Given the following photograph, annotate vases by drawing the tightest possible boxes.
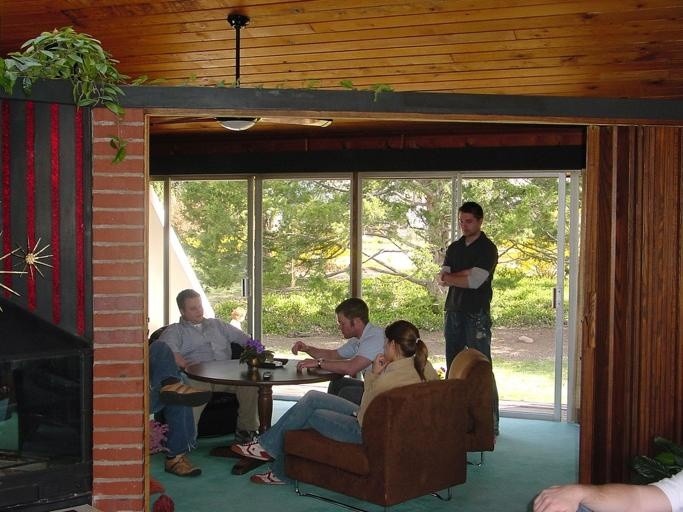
[249,358,259,366]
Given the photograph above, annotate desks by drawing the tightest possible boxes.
[184,358,344,475]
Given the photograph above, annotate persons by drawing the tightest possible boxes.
[157,289,261,444]
[149,340,212,477]
[531,469,682,512]
[229,320,441,485]
[291,298,385,405]
[438,202,499,436]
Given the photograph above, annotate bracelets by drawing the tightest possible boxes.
[442,272,449,281]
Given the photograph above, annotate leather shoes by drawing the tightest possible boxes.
[235,431,259,444]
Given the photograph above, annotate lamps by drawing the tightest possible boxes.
[214,117,261,131]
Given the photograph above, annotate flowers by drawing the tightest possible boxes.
[239,339,275,364]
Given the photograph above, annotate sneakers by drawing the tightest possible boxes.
[160,382,212,407]
[250,470,287,484]
[165,453,201,476]
[231,437,270,461]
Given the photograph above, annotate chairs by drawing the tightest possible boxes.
[283,379,468,511]
[149,326,239,438]
[447,349,496,466]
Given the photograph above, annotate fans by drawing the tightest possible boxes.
[150,116,334,129]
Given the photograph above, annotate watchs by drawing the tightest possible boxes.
[317,358,324,368]
